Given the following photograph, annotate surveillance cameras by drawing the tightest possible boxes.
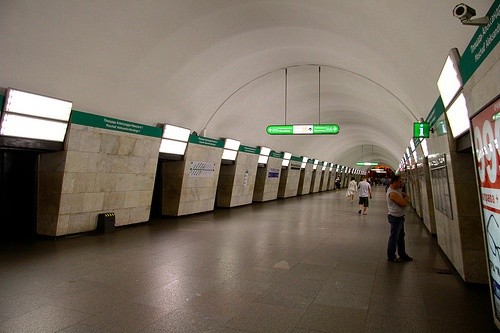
[452,3,477,20]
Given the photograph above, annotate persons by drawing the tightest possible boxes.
[367,177,385,186]
[347,178,358,203]
[386,175,413,262]
[335,176,341,194]
[357,176,372,216]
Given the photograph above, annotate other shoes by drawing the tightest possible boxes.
[358,210,361,214]
[363,213,367,215]
[399,255,413,261]
[387,258,402,264]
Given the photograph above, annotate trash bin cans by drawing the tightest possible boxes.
[97,213,115,235]
